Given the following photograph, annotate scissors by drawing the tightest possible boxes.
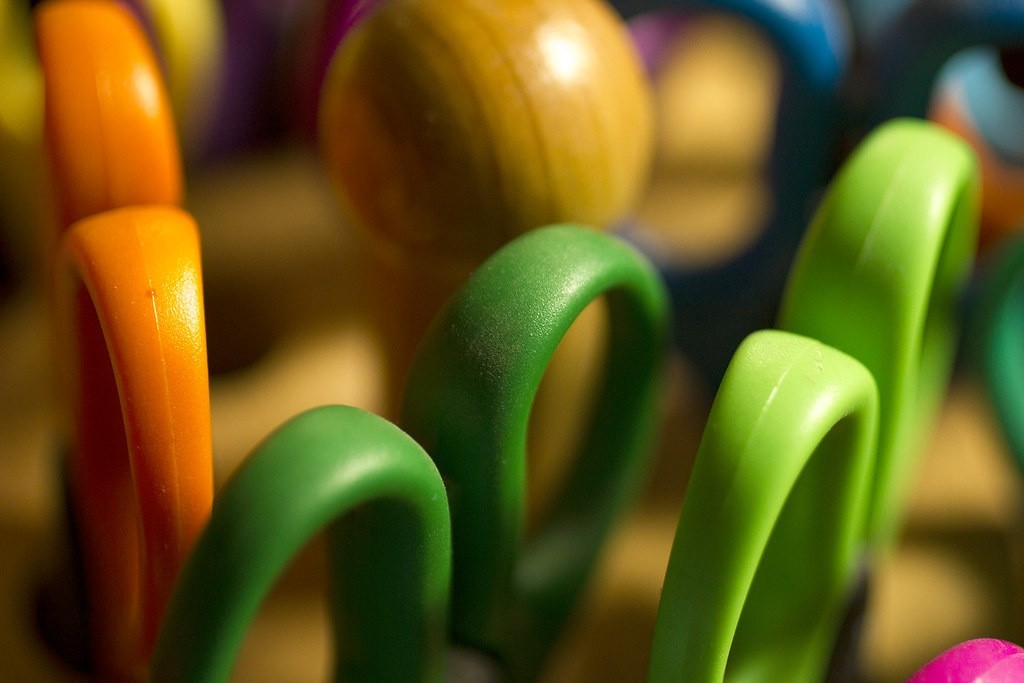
[30,0,218,683]
[149,223,675,683]
[647,122,985,683]
